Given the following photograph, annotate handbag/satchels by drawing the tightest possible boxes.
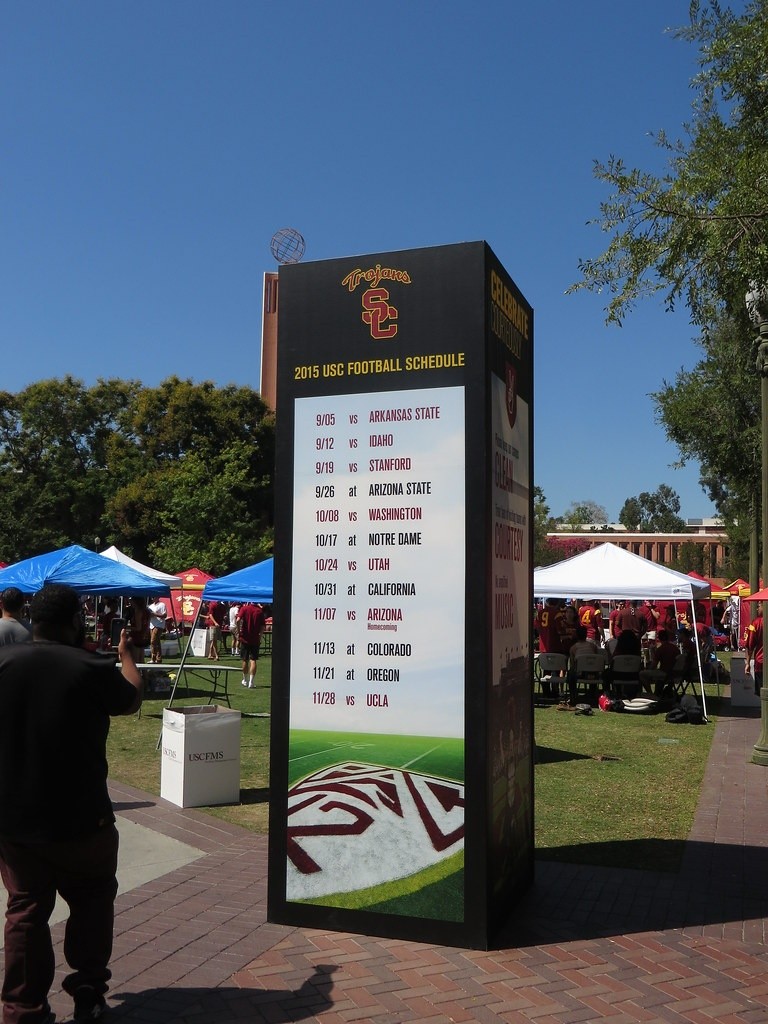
[665,704,708,724]
[711,663,731,685]
[132,632,151,648]
[598,691,627,713]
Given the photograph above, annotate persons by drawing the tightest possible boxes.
[197,600,271,656]
[77,596,135,648]
[0,584,145,1024]
[125,596,149,674]
[236,602,265,689]
[208,601,227,661]
[535,597,739,699]
[0,587,32,647]
[146,595,167,663]
[745,601,764,697]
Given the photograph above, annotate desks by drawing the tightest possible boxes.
[115,663,245,719]
[220,630,272,653]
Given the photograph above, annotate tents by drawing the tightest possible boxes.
[98,545,184,655]
[0,545,190,693]
[155,557,273,749]
[158,568,242,623]
[654,571,768,653]
[534,541,720,723]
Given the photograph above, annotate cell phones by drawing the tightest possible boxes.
[111,618,126,648]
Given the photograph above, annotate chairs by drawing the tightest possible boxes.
[659,655,686,705]
[609,654,641,699]
[676,655,702,704]
[574,654,605,699]
[538,653,568,702]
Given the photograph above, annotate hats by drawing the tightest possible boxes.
[681,693,701,709]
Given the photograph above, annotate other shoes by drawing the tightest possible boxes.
[208,656,213,658]
[231,654,235,656]
[248,684,256,688]
[147,659,155,663]
[216,656,220,661]
[73,996,106,1021]
[234,653,240,656]
[155,660,162,664]
[241,680,248,686]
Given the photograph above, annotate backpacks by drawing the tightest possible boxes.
[575,703,593,715]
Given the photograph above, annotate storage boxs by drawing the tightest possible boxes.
[149,676,171,692]
[144,642,178,657]
[160,704,242,809]
[190,629,211,657]
